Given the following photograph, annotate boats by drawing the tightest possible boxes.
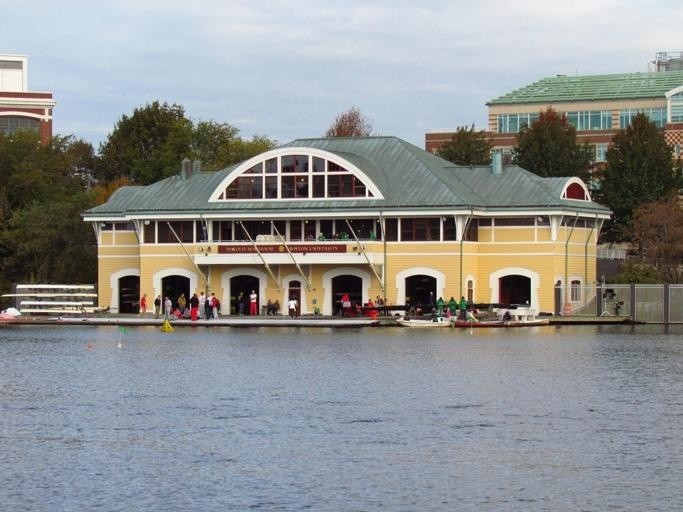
[393,315,452,327]
[495,307,551,327]
[453,318,505,328]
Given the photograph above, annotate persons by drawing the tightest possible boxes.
[449,297,457,316]
[316,232,325,241]
[341,293,351,307]
[428,291,436,312]
[238,291,245,316]
[250,290,258,315]
[334,231,349,241]
[367,229,375,241]
[304,230,314,241]
[138,294,148,319]
[459,296,468,321]
[266,300,280,316]
[501,310,513,327]
[288,296,298,318]
[368,295,384,307]
[155,288,220,320]
[438,297,445,313]
